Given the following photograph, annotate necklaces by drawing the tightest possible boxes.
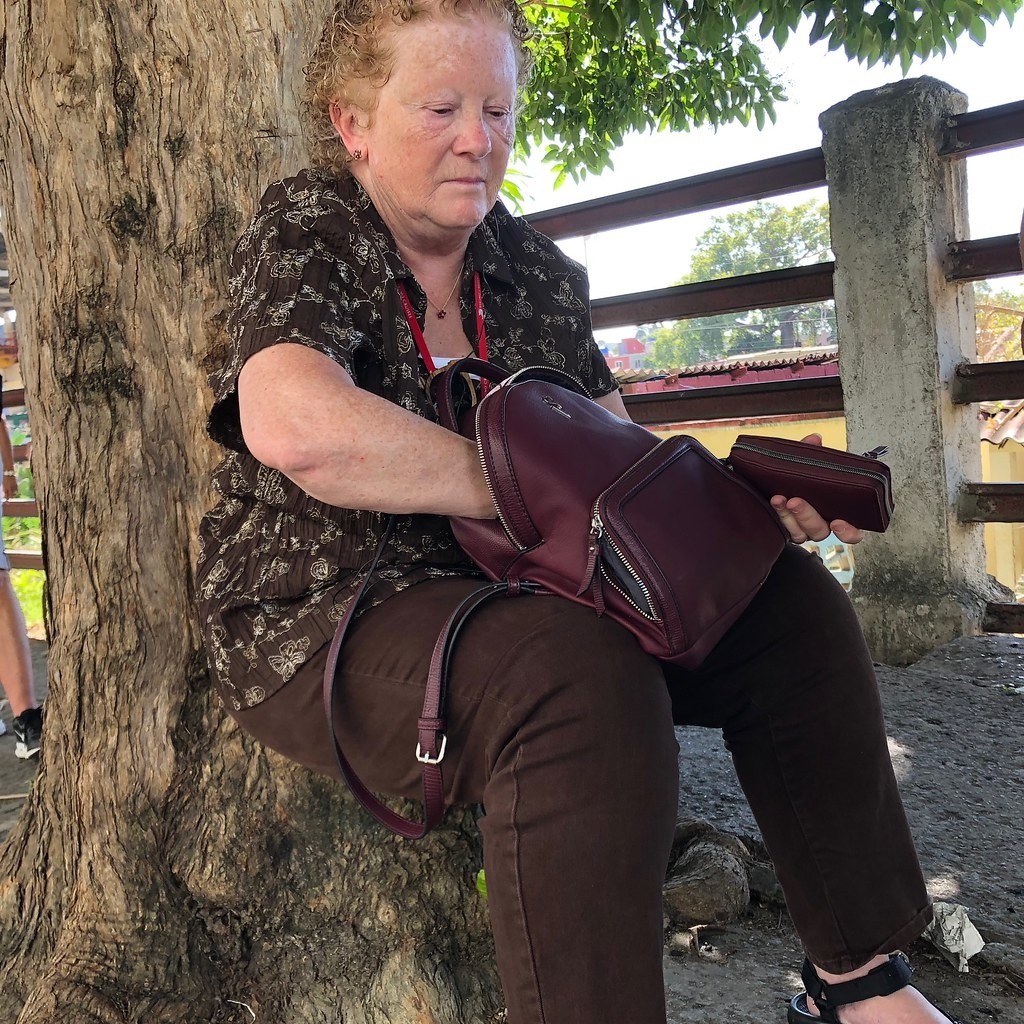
[426,258,466,318]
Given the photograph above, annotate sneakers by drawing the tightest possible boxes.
[0,719,6,736]
[12,704,44,759]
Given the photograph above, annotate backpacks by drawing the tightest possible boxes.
[330,361,789,836]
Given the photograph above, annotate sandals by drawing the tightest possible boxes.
[787,951,960,1024]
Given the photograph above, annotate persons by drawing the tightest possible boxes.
[194,0,965,1024]
[0,371,43,759]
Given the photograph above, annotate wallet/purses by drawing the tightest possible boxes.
[730,435,896,534]
